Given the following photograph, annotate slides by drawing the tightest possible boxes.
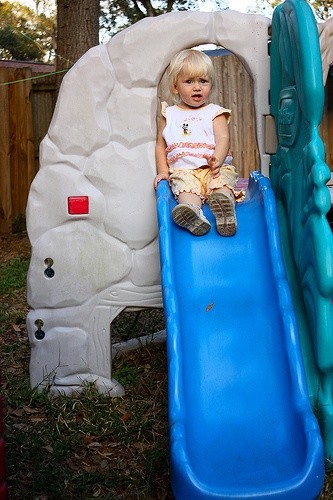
[155,171,326,500]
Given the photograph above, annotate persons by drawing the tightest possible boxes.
[153,48,241,237]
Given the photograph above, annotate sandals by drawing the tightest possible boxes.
[172,202,212,236]
[210,190,237,236]
